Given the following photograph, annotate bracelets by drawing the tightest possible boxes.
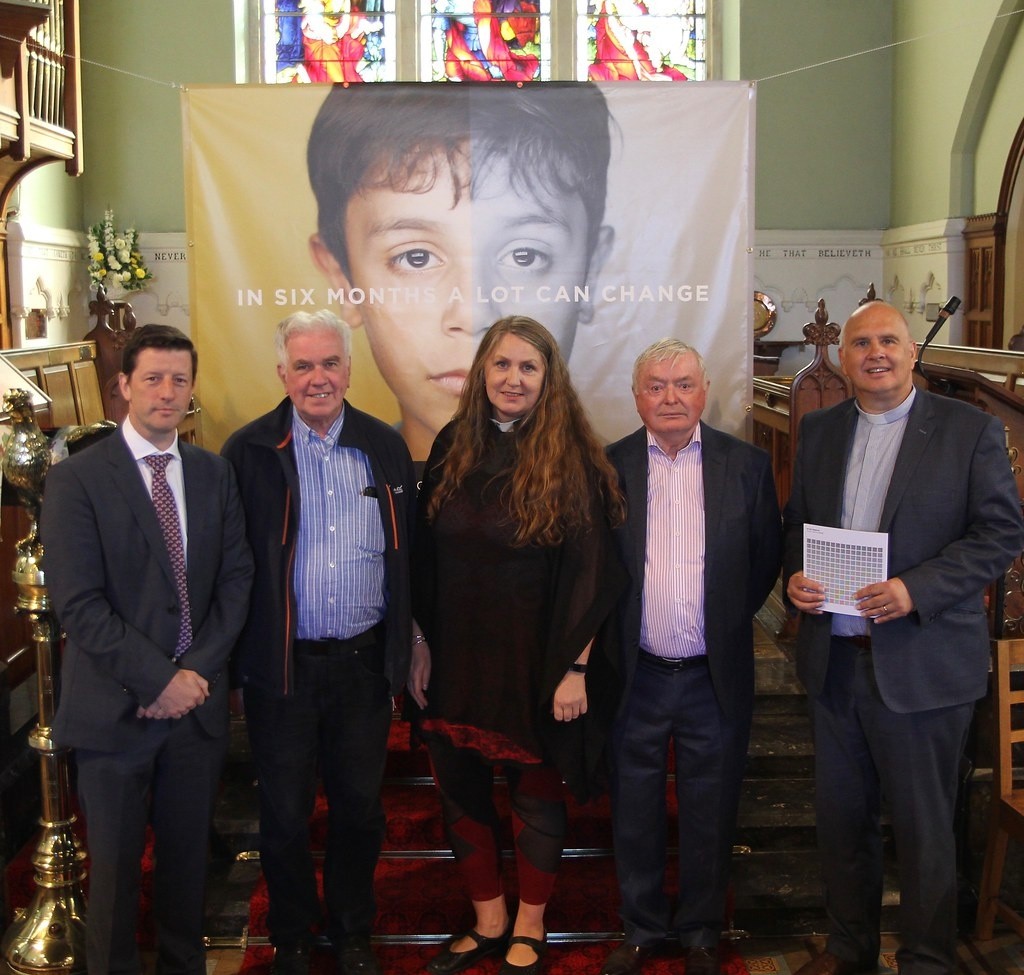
[411,635,426,646]
[568,661,589,673]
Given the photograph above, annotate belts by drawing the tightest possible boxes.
[847,635,871,649]
[639,649,709,671]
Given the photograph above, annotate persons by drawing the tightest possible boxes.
[307,81,615,463]
[595,337,784,975]
[782,300,1023,975]
[409,316,625,975]
[220,309,417,975]
[42,323,250,975]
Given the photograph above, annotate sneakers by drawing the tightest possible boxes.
[685,946,721,975]
[600,939,664,975]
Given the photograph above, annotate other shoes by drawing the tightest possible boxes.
[501,929,548,975]
[425,917,509,975]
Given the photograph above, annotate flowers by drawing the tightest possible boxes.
[87,203,155,298]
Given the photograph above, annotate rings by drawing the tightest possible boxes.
[883,606,889,613]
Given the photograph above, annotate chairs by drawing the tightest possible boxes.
[973,637,1024,946]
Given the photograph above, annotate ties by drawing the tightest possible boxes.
[143,452,193,659]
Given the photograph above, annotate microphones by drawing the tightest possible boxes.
[918,295,961,389]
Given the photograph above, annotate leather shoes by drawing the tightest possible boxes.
[330,934,381,974]
[271,938,312,975]
[794,950,880,975]
